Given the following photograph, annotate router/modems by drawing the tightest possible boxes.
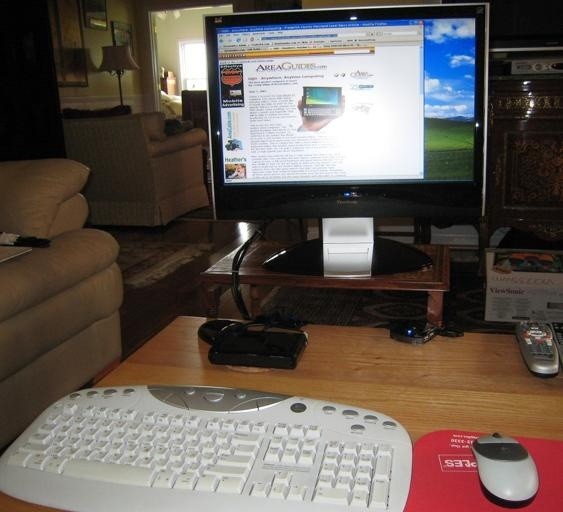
[209,329,307,370]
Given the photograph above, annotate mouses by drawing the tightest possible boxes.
[473,431,539,508]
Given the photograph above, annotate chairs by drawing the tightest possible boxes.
[178,88,210,190]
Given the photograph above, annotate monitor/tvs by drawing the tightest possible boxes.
[203,3,490,278]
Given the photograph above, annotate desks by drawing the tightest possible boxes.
[0,313,562,512]
[194,232,454,331]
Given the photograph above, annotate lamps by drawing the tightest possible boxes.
[95,42,141,116]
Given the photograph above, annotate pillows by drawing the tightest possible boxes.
[59,104,132,118]
[164,119,194,138]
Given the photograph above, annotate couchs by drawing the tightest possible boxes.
[57,109,211,233]
[0,151,127,459]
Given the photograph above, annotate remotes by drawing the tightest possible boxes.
[513,318,563,377]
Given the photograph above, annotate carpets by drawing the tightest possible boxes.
[247,248,562,334]
[100,228,253,363]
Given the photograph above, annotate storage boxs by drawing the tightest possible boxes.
[478,245,562,325]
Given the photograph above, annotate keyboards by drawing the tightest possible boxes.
[1,384,413,511]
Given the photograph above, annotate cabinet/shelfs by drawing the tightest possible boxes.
[160,70,175,96]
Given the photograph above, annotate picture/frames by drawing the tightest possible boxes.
[109,19,134,57]
[52,0,88,88]
[82,0,108,31]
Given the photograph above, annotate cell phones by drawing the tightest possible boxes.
[301,85,342,116]
